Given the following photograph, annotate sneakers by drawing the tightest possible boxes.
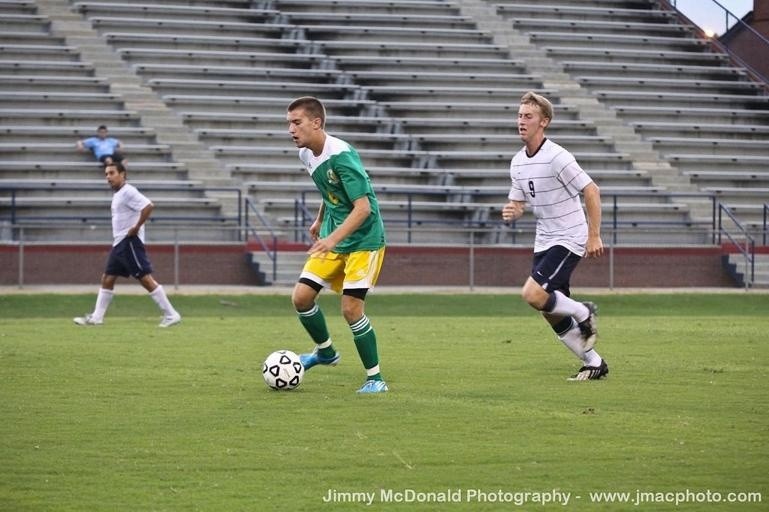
[578,301,598,353]
[566,359,608,381]
[158,312,181,327]
[356,380,388,394]
[300,352,340,371]
[73,316,104,325]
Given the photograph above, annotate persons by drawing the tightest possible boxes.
[76,125,128,166]
[72,161,182,329]
[501,91,610,382]
[285,95,388,392]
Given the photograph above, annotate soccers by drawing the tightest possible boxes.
[262,350,304,390]
